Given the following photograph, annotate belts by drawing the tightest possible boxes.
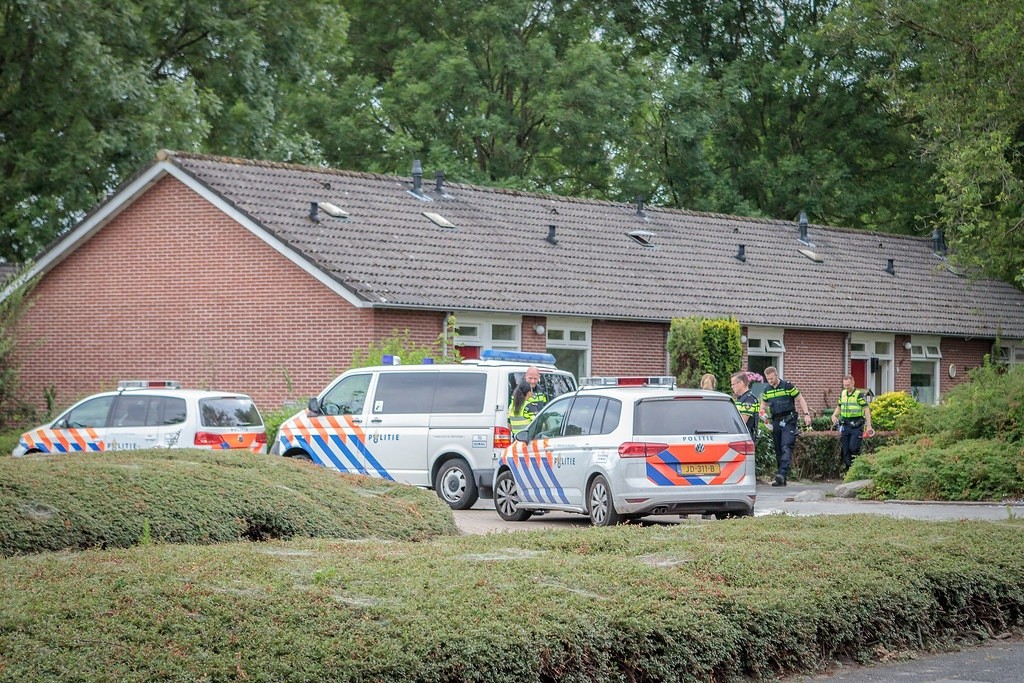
[773,414,787,417]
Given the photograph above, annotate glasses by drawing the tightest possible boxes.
[730,381,741,386]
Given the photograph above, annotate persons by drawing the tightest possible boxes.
[831,375,876,475]
[507,367,550,516]
[700,366,811,486]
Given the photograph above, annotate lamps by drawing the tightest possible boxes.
[902,341,912,349]
[533,321,545,336]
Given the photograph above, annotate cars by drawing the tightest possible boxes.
[489,375,758,528]
[10,379,268,457]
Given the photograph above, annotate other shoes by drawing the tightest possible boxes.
[774,474,787,484]
[772,480,786,486]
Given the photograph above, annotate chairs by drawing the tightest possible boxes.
[121,404,145,425]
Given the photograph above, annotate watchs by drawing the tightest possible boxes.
[805,412,810,416]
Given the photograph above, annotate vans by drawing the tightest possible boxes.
[264,346,579,510]
